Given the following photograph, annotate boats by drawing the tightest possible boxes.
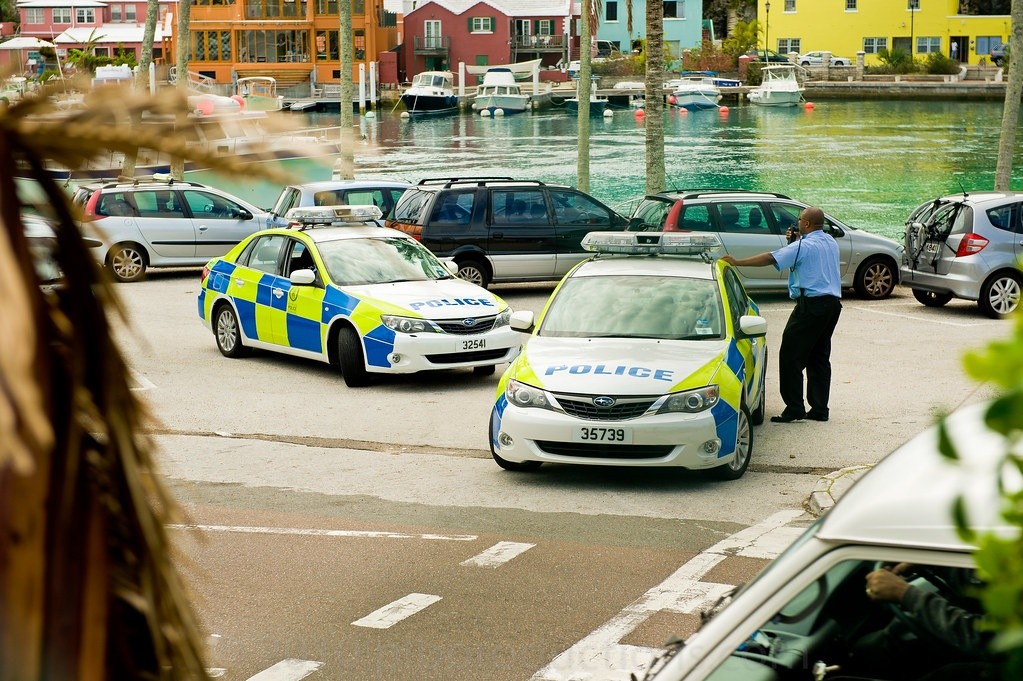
[746,64,807,108]
[466,58,543,80]
[563,74,610,114]
[14,91,342,184]
[473,67,534,117]
[397,71,474,116]
[560,40,632,75]
[672,76,723,110]
[0,24,91,124]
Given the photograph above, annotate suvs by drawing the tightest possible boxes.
[382,176,630,289]
[989,42,1012,68]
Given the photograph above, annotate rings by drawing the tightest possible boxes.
[866,589,872,592]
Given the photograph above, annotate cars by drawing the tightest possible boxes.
[897,187,1023,320]
[18,212,74,288]
[746,48,790,63]
[271,180,416,228]
[66,174,294,284]
[197,203,522,389]
[621,187,903,301]
[798,51,853,65]
[489,230,772,480]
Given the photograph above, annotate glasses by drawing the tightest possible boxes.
[798,216,806,221]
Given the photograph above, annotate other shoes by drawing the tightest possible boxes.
[804,407,828,421]
[770,404,804,422]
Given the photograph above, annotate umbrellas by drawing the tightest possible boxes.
[0,37,58,76]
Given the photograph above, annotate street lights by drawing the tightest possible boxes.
[765,0,770,49]
[910,0,916,59]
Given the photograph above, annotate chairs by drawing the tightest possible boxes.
[721,204,739,227]
[173,194,181,211]
[509,199,527,221]
[749,208,762,229]
[530,203,545,221]
[157,195,169,212]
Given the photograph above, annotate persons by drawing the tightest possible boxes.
[722,207,842,423]
[951,38,958,59]
[865,562,995,651]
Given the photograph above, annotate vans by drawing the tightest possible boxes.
[628,397,1023,681]
